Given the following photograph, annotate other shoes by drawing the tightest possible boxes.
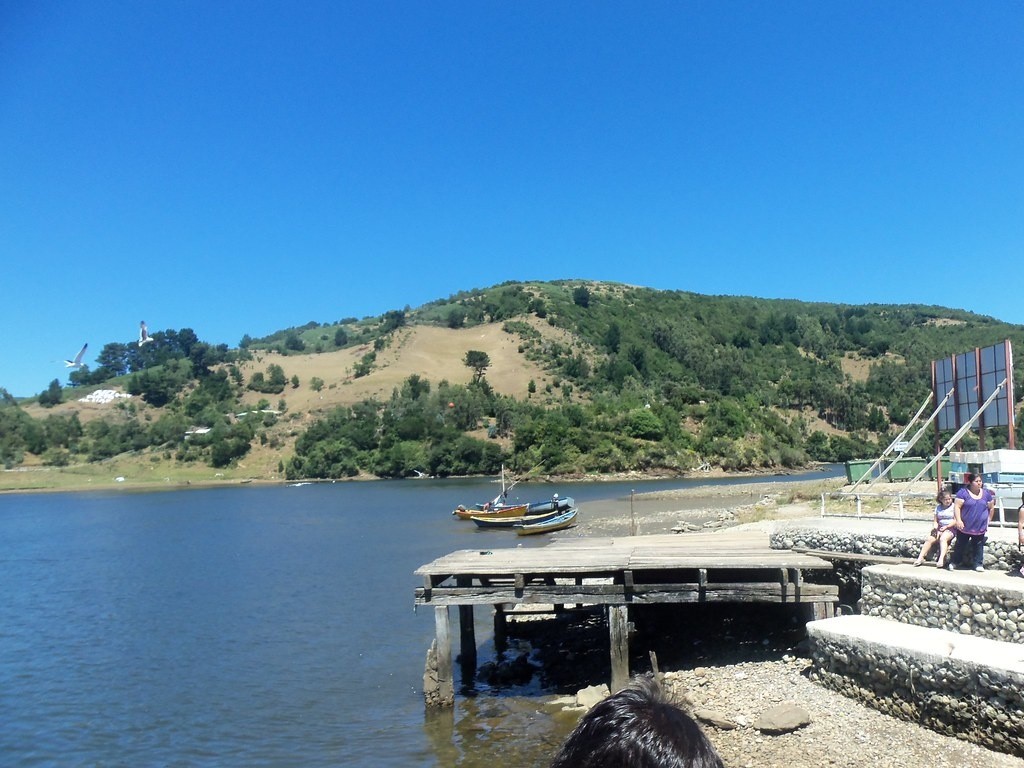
[974,566,985,571]
[915,556,925,565]
[937,557,948,567]
[948,562,959,570]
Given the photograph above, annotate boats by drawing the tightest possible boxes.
[452,459,575,520]
[513,508,578,536]
[470,510,558,527]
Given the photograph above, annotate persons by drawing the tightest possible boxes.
[482,502,493,512]
[947,473,995,571]
[1018,492,1024,578]
[913,490,958,567]
[550,675,724,768]
